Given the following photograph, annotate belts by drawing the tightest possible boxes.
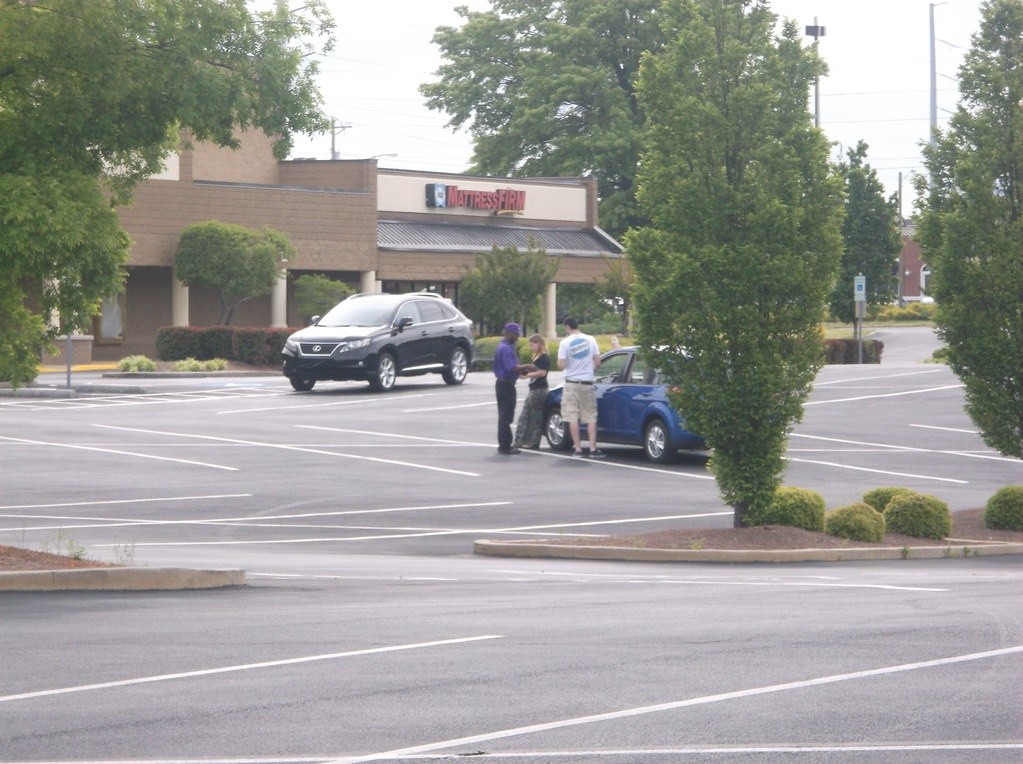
[566,380,593,385]
[499,378,515,383]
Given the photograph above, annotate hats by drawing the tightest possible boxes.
[505,322,523,335]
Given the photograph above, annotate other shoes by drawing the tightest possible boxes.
[572,452,584,458]
[522,443,538,449]
[589,449,607,459]
[498,446,520,454]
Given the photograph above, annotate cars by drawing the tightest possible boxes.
[542,343,728,466]
[895,296,932,306]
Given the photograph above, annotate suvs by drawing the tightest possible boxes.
[281,291,474,393]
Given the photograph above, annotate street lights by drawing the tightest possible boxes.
[806,15,827,129]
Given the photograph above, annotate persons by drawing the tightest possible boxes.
[515,333,550,448]
[557,316,608,459]
[493,323,537,454]
